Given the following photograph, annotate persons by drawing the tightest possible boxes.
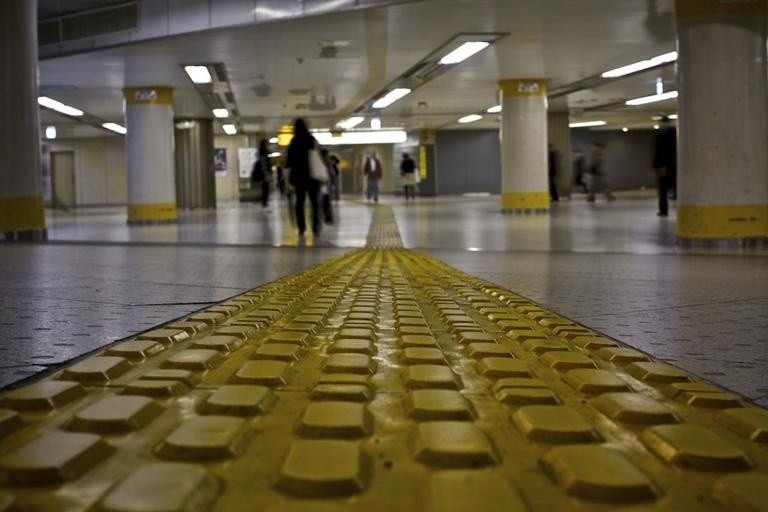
[361,148,383,201]
[569,148,588,194]
[398,154,418,200]
[648,113,677,217]
[548,141,572,203]
[214,150,226,171]
[251,114,340,237]
[585,140,616,203]
